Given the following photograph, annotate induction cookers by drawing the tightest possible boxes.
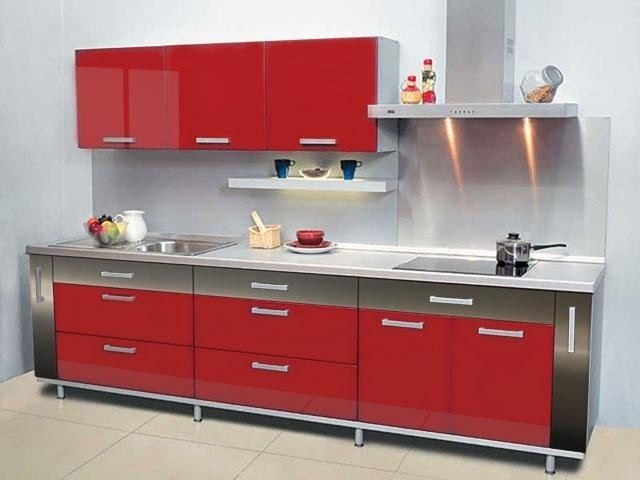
[394,256,539,278]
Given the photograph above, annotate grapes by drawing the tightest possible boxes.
[98,213,112,224]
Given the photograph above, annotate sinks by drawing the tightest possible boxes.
[134,237,223,254]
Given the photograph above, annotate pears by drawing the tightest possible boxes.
[99,221,128,243]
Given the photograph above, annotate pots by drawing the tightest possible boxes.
[497,232,566,264]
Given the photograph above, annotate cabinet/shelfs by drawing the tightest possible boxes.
[162,38,268,150]
[192,255,360,450]
[30,254,194,428]
[267,36,378,150]
[76,43,166,151]
[358,269,591,459]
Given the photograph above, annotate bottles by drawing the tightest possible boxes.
[519,66,564,103]
[399,59,438,105]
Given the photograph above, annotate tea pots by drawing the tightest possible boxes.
[115,211,148,242]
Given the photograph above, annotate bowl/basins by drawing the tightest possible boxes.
[296,230,324,245]
[83,220,128,247]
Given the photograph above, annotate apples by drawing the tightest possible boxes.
[87,219,102,234]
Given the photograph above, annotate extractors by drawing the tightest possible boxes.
[368,0,579,121]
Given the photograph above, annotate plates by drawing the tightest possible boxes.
[283,241,335,253]
[297,169,331,180]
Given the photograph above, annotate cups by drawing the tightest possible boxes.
[273,159,295,178]
[340,160,361,181]
